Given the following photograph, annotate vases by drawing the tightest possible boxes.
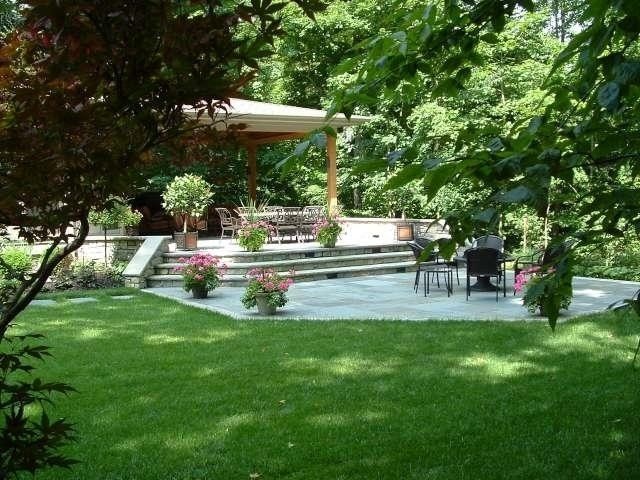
[320,239,335,248]
[252,290,278,314]
[189,284,209,298]
[247,247,259,252]
[539,306,559,316]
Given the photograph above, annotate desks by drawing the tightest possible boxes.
[452,256,515,292]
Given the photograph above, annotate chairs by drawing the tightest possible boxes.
[214,205,327,244]
[464,246,506,304]
[513,248,546,295]
[471,235,505,284]
[407,237,460,298]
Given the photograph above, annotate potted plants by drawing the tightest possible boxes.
[160,172,216,250]
[120,206,144,236]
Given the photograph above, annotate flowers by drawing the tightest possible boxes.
[240,266,295,311]
[172,250,228,293]
[312,213,350,239]
[513,267,573,313]
